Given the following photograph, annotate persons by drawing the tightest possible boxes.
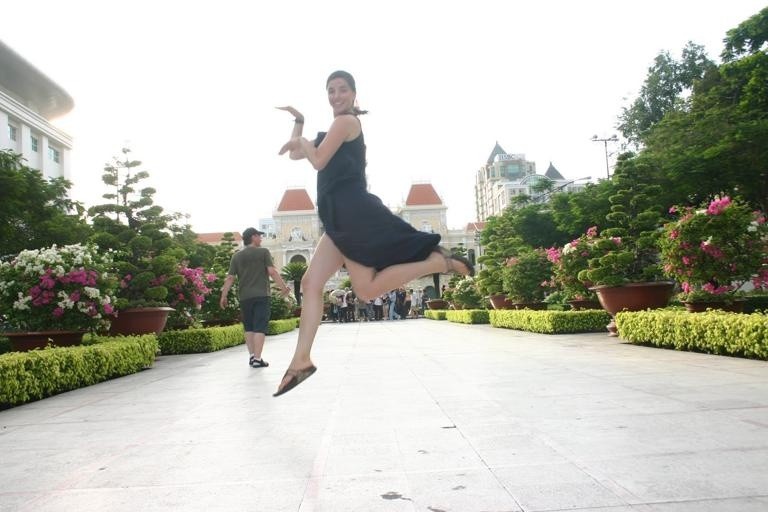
[323,284,449,323]
[219,227,294,369]
[270,67,475,399]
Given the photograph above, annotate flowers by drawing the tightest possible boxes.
[541,226,622,301]
[657,192,768,303]
[122,265,216,323]
[0,243,131,333]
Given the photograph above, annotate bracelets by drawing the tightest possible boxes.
[294,118,304,124]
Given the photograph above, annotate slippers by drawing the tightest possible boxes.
[431,245,476,277]
[271,364,320,398]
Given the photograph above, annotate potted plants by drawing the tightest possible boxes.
[281,262,309,317]
[578,183,676,336]
[86,149,177,339]
[424,247,467,309]
[202,256,240,329]
[478,220,528,309]
[502,254,555,310]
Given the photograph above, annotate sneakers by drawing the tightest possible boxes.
[249,356,269,368]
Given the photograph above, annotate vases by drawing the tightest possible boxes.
[680,300,748,313]
[565,300,602,310]
[2,331,90,351]
[164,322,191,330]
